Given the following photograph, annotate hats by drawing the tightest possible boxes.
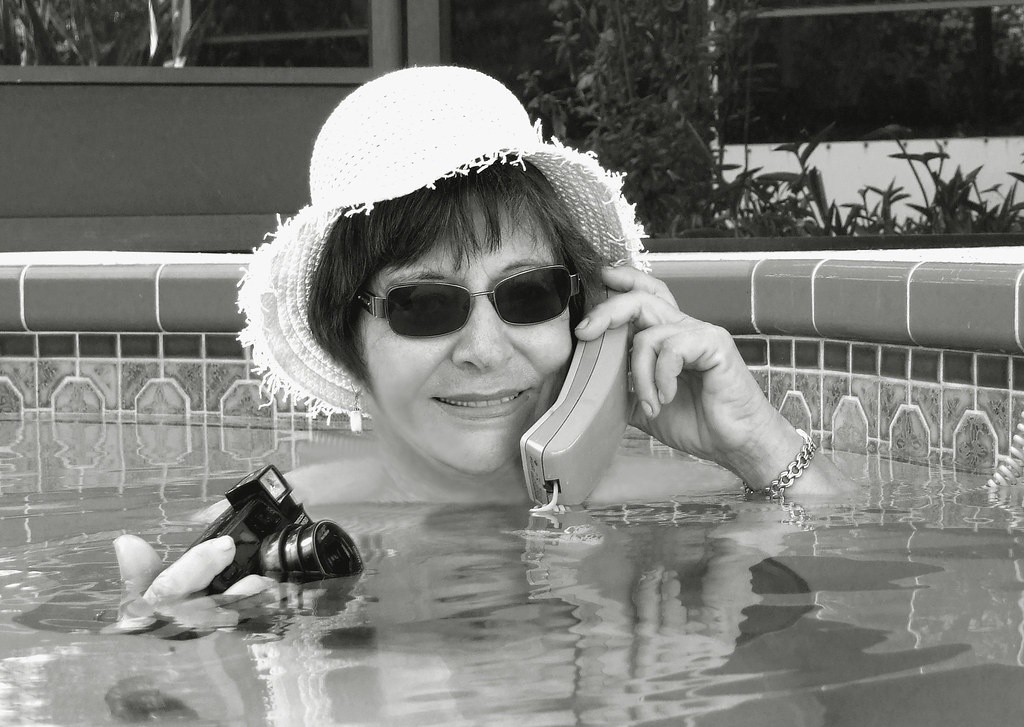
[235,63,653,432]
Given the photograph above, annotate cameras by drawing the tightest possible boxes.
[185,464,364,596]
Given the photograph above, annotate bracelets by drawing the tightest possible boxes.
[746,425,814,501]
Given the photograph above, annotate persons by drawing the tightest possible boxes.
[115,64,869,630]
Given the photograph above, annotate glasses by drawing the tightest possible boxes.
[354,262,580,339]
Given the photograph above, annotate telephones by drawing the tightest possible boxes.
[519,244,640,511]
